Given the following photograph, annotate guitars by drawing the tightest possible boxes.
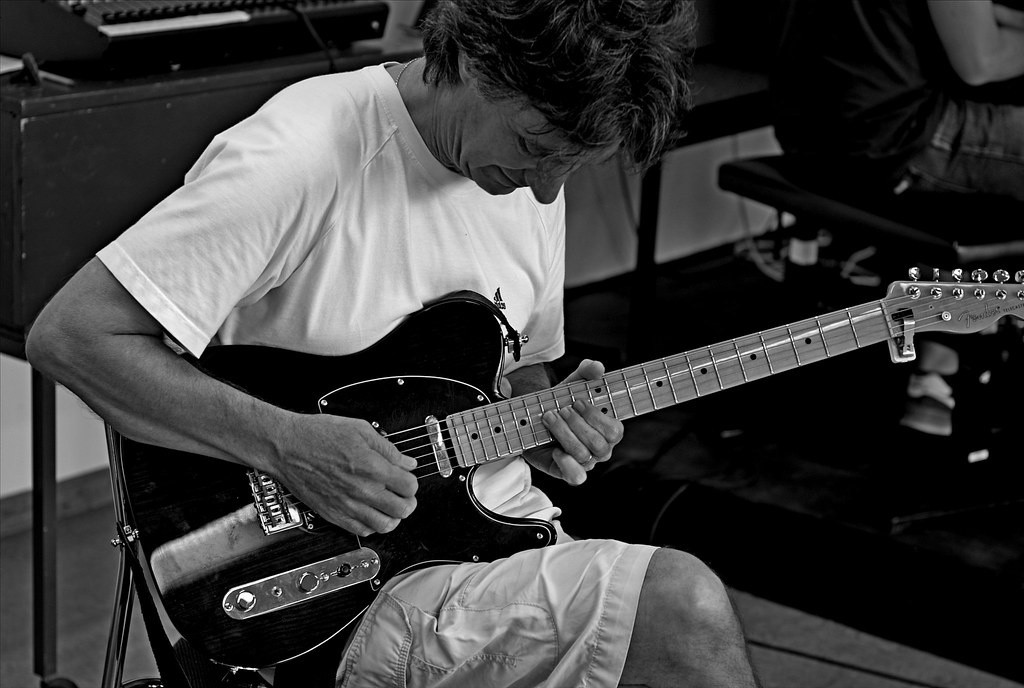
[115,265,1023,671]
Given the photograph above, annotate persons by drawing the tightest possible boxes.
[24,1,755,687]
[766,1,1024,437]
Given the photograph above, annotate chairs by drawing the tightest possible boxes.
[105,410,281,688]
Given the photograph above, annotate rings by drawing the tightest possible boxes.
[580,453,593,466]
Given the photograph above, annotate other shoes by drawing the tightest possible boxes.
[900,371,956,435]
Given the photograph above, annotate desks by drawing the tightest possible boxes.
[717,155,1024,321]
[0,20,429,688]
